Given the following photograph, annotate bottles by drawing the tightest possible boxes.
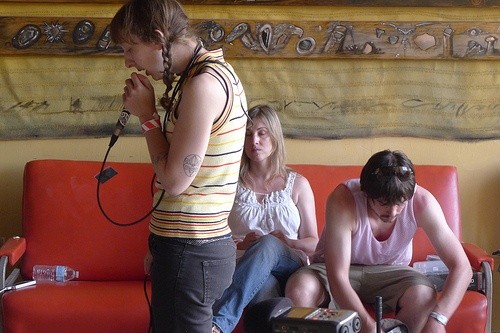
[33,265,80,283]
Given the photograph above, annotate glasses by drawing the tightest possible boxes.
[366,163,415,182]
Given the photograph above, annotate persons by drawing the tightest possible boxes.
[285,150,473,333]
[211,104,319,333]
[110,0,247,333]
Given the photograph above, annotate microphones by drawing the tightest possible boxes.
[108,106,131,147]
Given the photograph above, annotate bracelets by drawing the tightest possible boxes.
[141,112,162,135]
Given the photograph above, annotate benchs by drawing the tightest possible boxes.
[0,160,494,333]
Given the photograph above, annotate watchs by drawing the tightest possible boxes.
[430,312,449,326]
